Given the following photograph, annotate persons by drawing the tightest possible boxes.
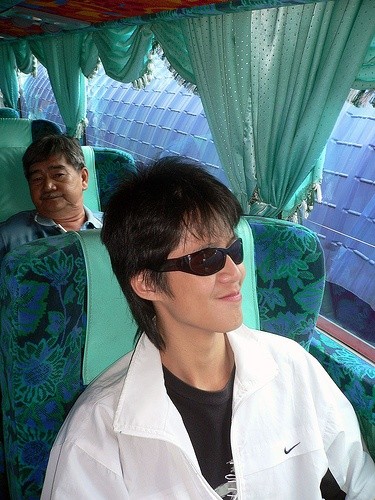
[0,136,104,257]
[39,155,375,500]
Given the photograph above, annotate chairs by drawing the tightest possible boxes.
[0,147,138,224]
[0,216,375,500]
[0,107,19,118]
[0,118,33,147]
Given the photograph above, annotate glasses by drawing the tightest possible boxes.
[142,237,244,276]
